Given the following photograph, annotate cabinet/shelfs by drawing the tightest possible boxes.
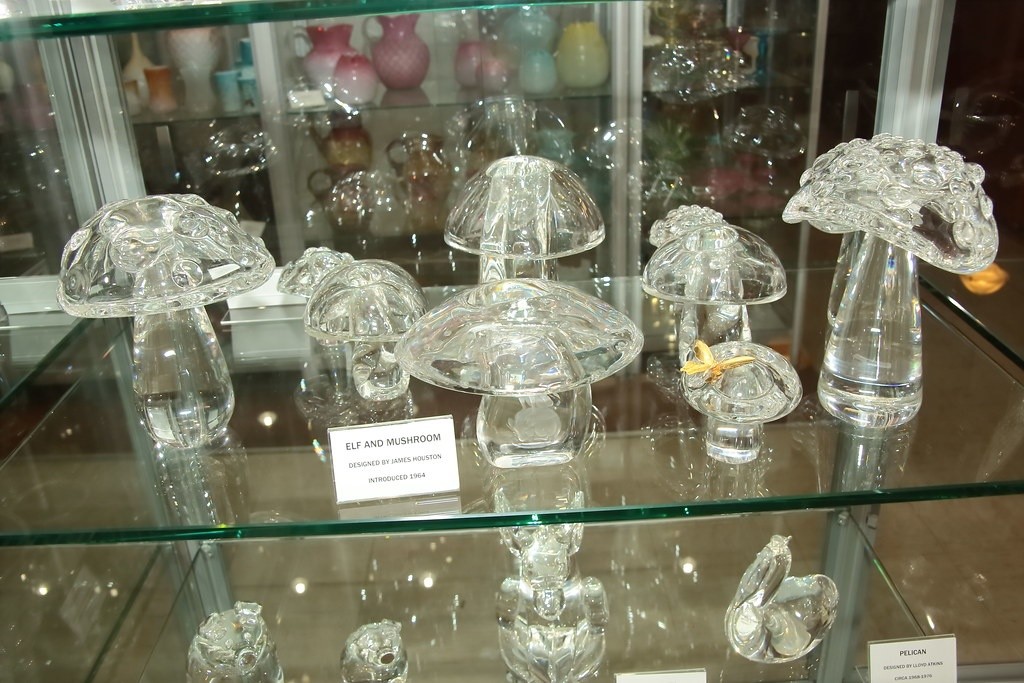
[0,0,1024,683]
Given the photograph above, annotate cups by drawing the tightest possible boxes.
[143,66,178,115]
[238,74,259,108]
[213,71,244,111]
